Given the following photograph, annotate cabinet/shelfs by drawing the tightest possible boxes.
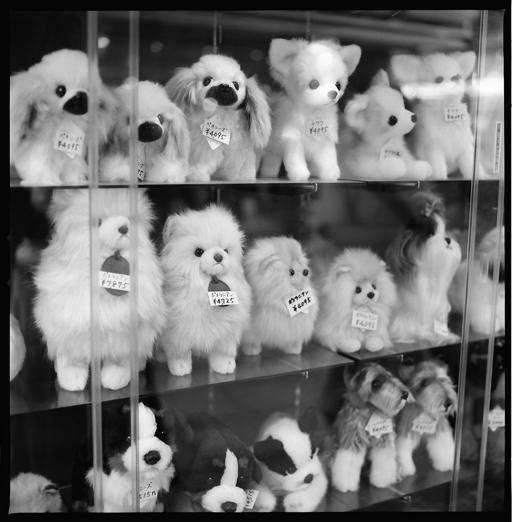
[10,167,507,513]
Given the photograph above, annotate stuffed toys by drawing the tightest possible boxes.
[258,36,362,183]
[389,49,488,181]
[9,48,120,188]
[308,182,506,352]
[164,53,273,183]
[150,202,252,378]
[32,186,167,392]
[336,67,433,181]
[97,79,191,184]
[239,233,320,356]
[323,341,507,493]
[465,51,506,180]
[6,397,177,513]
[166,404,330,512]
